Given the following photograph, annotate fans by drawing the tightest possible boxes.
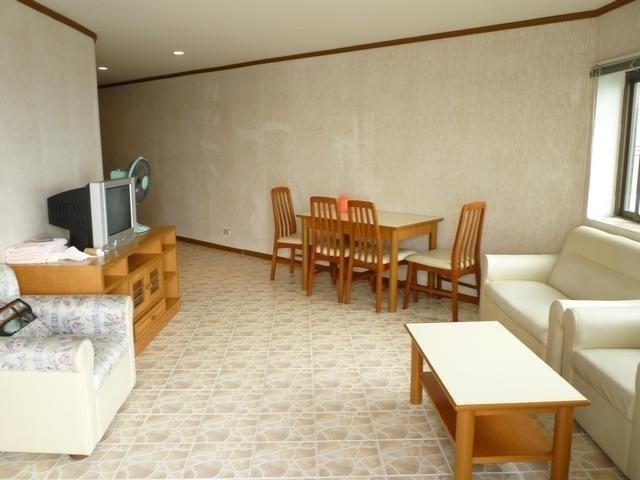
[110,156,151,234]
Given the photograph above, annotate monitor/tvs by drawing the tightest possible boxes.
[47,176,136,255]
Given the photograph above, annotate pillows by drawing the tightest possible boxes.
[0,300,53,339]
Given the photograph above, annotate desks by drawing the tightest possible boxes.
[296,210,444,313]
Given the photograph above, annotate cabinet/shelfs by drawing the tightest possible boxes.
[11,224,179,357]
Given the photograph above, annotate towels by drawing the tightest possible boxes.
[1,237,68,264]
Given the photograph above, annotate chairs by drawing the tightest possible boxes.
[480,226,640,375]
[346,200,419,313]
[0,264,138,457]
[403,202,486,322]
[562,306,640,480]
[270,186,334,291]
[308,196,373,303]
